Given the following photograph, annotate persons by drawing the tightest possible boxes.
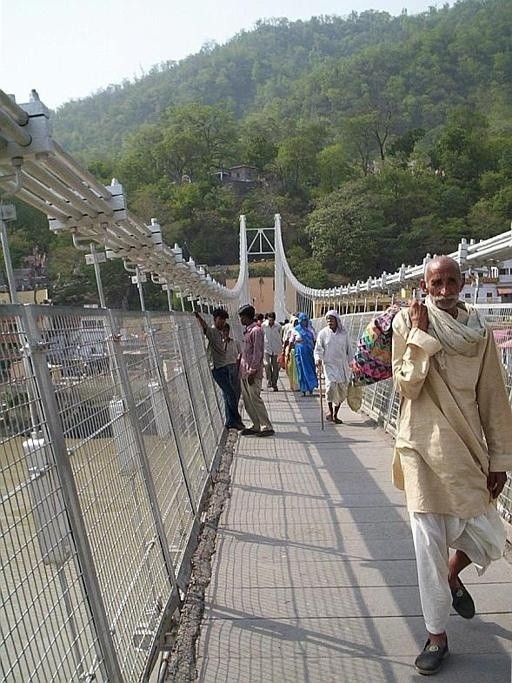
[314,310,354,424]
[391,257,512,675]
[193,304,317,438]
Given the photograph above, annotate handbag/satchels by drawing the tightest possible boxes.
[352,300,411,388]
[347,381,362,412]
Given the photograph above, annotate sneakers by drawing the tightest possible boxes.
[267,380,272,388]
[241,426,260,435]
[255,430,275,436]
[415,633,449,677]
[326,414,343,424]
[227,421,245,431]
[274,386,278,391]
[449,575,475,618]
